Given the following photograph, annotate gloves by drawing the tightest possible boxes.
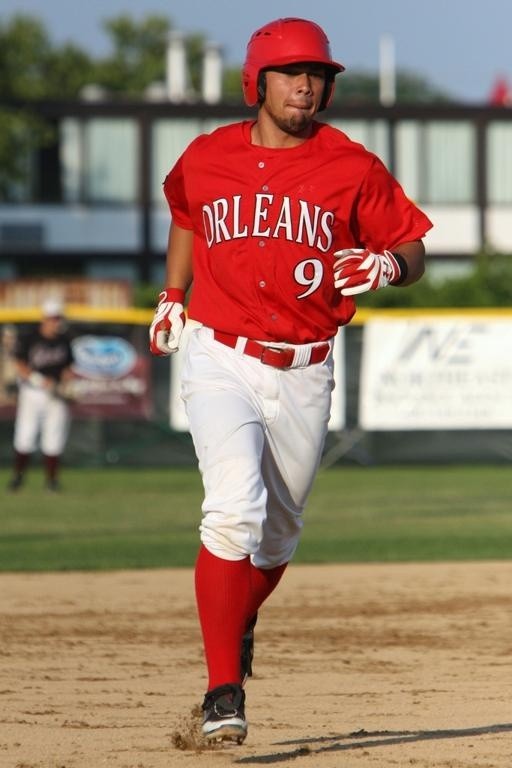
[149,288,185,357]
[333,248,407,296]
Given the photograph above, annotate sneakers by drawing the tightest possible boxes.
[240,612,257,687]
[201,683,247,745]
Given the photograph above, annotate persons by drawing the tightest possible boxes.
[8,301,77,496]
[145,15,433,747]
[0,323,24,407]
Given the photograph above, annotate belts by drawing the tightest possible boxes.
[214,330,329,367]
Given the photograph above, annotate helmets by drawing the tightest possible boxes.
[242,18,345,112]
